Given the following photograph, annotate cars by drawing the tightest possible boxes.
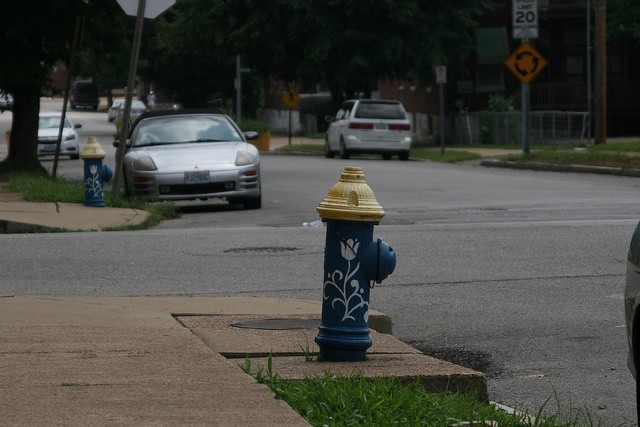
[0,93,13,105]
[114,100,146,121]
[108,99,124,121]
[122,113,261,210]
[113,106,228,201]
[625,219,640,426]
[36,112,81,159]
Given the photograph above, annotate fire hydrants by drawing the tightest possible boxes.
[315,165,397,362]
[80,135,113,206]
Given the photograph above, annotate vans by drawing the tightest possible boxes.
[69,80,98,110]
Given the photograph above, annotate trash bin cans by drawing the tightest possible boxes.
[259,128,270,151]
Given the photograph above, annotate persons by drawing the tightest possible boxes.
[147,90,156,111]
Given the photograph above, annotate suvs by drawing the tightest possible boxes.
[323,99,412,157]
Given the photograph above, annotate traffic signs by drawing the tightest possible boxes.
[513,0,538,37]
